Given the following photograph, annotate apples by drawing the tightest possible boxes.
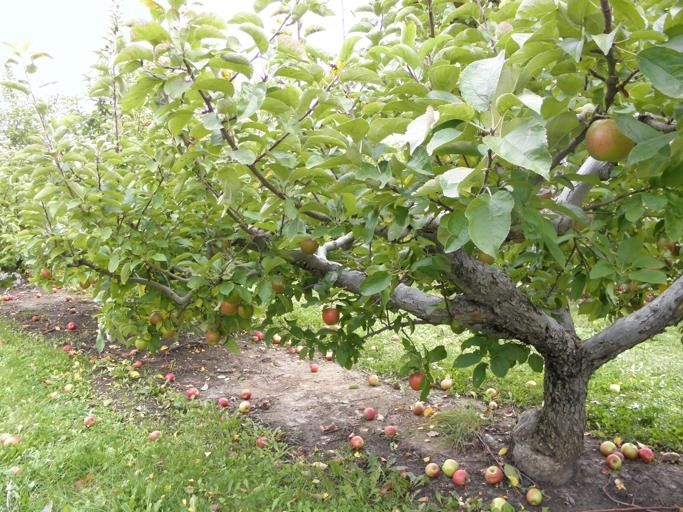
[0,108,683,512]
